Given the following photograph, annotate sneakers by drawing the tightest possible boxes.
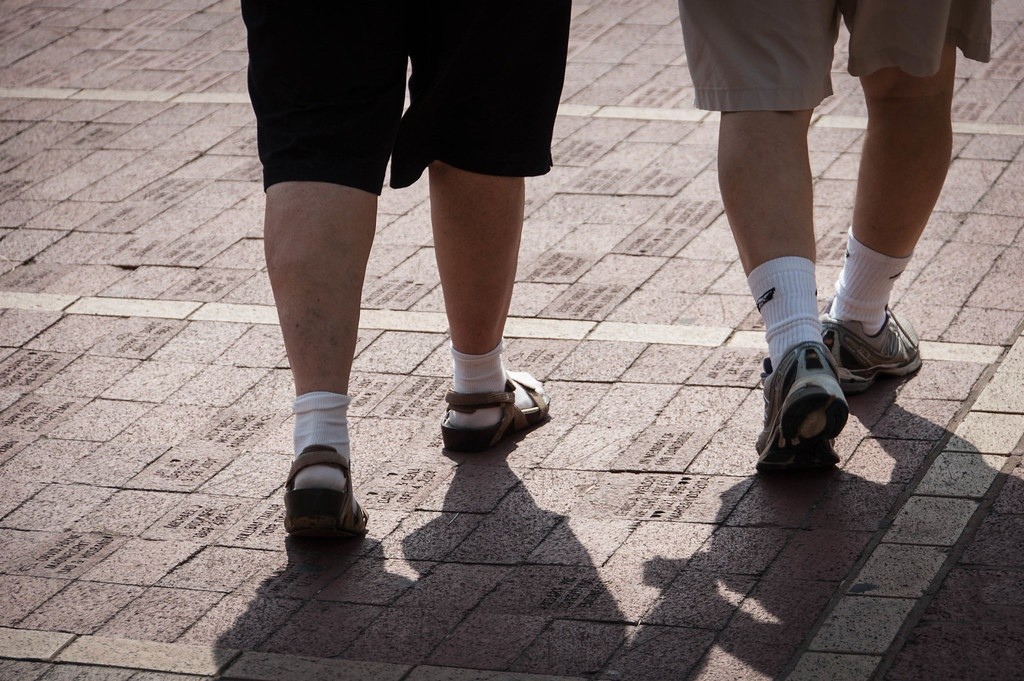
[819,303,922,392]
[756,341,850,478]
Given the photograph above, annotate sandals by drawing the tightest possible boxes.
[284,451,369,534]
[441,371,551,451]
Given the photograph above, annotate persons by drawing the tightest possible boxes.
[677,1,994,481]
[242,1,575,538]
